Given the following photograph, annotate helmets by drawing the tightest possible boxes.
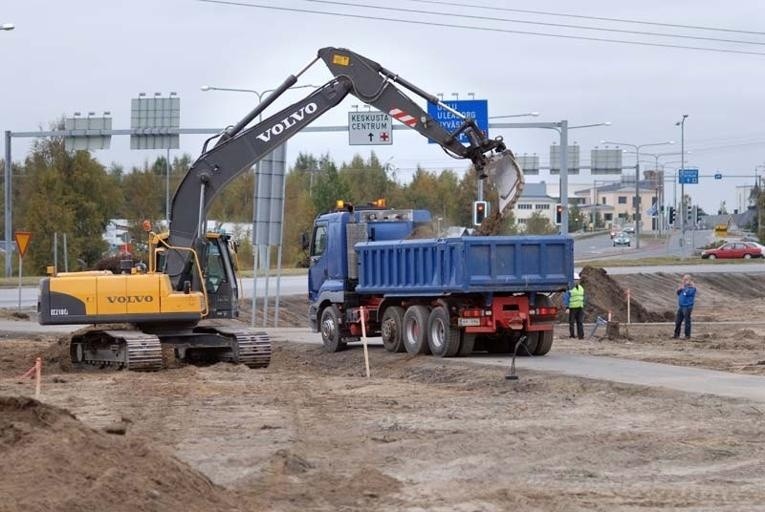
[573,272,581,280]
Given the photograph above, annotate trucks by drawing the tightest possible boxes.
[297,196,575,359]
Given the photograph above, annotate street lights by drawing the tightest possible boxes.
[200,84,330,127]
[1,23,16,36]
[600,139,674,248]
[567,121,611,128]
[622,149,693,237]
[674,113,690,260]
[486,112,541,121]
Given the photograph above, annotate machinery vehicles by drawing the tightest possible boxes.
[38,44,524,371]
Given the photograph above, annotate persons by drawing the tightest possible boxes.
[563,272,588,341]
[668,273,697,340]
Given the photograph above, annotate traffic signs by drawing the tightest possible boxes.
[425,100,490,145]
[347,111,394,145]
[678,168,698,183]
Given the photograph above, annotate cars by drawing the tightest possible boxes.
[701,241,765,259]
[606,221,638,250]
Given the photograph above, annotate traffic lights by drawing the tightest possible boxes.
[684,204,691,223]
[475,199,485,225]
[555,204,561,224]
[693,205,703,225]
[667,205,675,225]
